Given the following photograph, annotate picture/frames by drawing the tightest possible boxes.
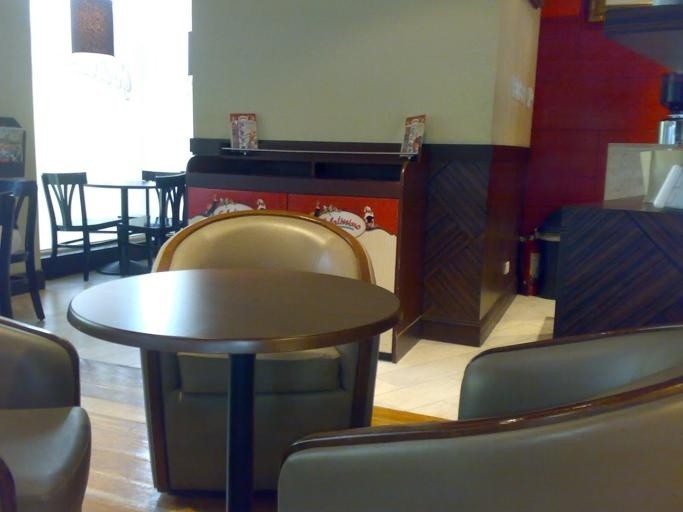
[0,117,26,177]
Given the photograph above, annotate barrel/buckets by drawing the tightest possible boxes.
[657,72,683,114]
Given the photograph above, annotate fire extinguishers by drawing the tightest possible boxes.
[521,235,541,297]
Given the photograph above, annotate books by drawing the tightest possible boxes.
[228,112,258,149]
[0,126,25,166]
[398,113,426,157]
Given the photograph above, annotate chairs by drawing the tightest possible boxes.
[0,316,90,512]
[0,171,187,322]
[139,210,379,495]
[275,324,683,512]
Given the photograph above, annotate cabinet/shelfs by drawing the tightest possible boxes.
[186,147,425,362]
